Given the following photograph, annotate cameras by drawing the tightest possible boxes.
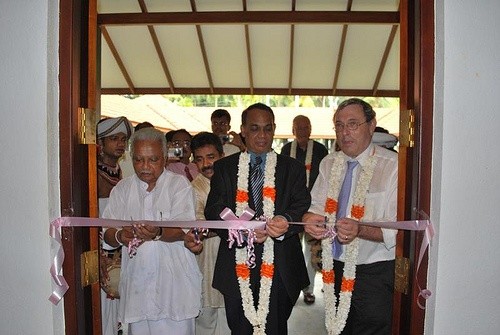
[168,148,183,157]
[219,134,229,143]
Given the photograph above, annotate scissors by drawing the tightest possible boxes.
[323,216,327,228]
[193,225,200,242]
[131,216,133,226]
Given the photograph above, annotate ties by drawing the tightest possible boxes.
[250,156,264,217]
[184,167,193,182]
[332,161,359,258]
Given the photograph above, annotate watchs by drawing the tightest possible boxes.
[152,226,163,241]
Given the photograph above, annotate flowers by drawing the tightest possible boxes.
[235,150,277,335]
[321,146,376,335]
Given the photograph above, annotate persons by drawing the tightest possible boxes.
[204,103,312,335]
[98,108,398,335]
[301,96,399,335]
[101,128,203,335]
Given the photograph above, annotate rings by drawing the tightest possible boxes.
[345,235,348,240]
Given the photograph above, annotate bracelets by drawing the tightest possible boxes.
[114,229,123,246]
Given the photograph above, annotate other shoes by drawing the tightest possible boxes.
[304,293,315,303]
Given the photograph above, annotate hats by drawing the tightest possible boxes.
[97,116,134,140]
